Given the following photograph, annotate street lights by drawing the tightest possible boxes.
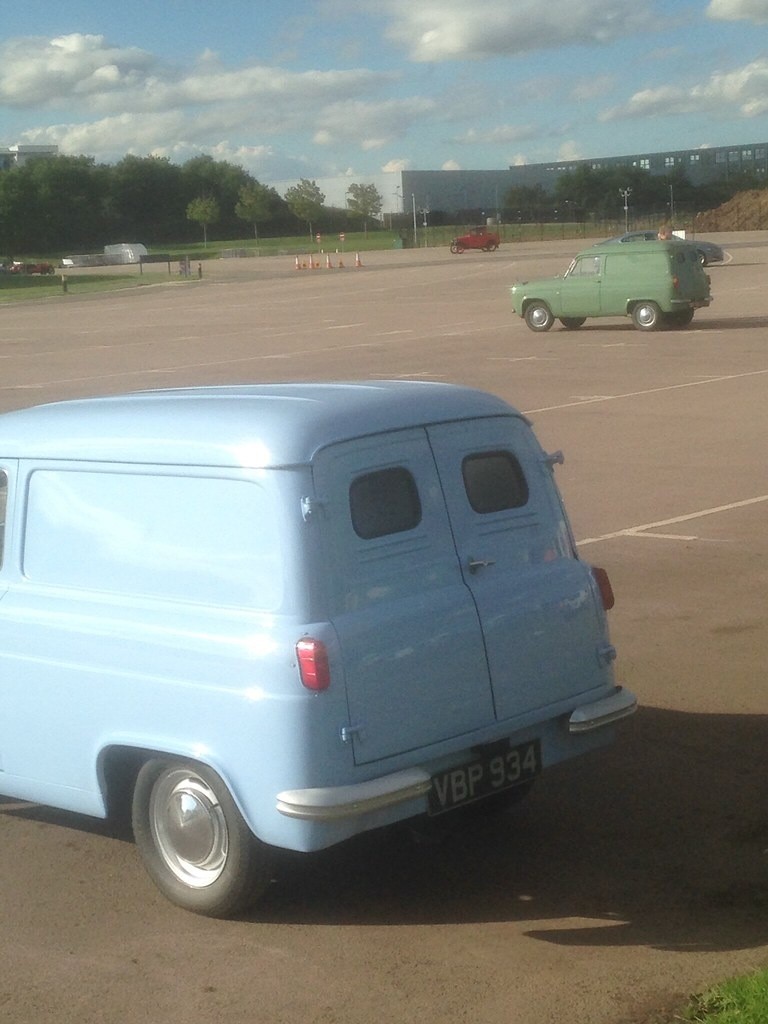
[619,188,633,232]
[420,209,431,248]
[670,185,673,225]
[410,193,416,246]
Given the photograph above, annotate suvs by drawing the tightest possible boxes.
[509,239,712,333]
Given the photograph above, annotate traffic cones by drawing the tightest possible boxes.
[307,255,314,268]
[354,252,362,267]
[296,255,301,269]
[325,255,333,269]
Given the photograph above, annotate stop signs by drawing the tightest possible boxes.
[316,233,321,240]
[339,232,345,239]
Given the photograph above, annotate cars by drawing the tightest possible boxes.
[10,263,53,274]
[595,231,724,269]
[451,226,500,255]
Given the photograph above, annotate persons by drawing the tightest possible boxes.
[658,224,672,241]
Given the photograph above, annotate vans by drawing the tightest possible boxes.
[0,380,639,920]
